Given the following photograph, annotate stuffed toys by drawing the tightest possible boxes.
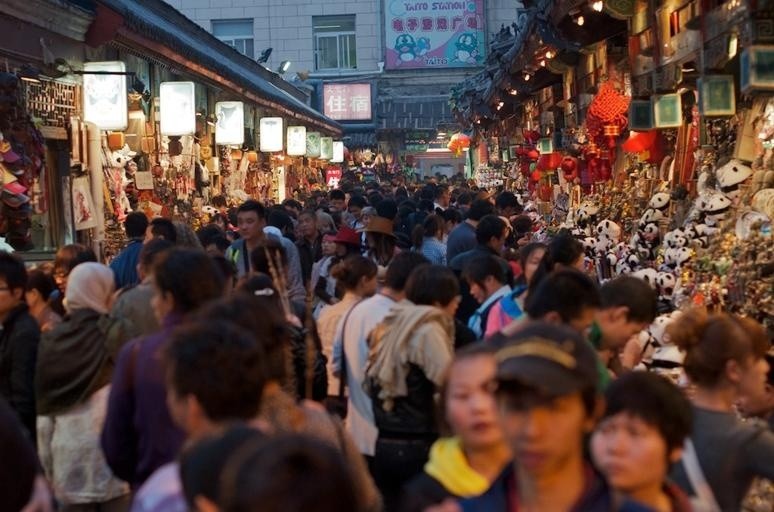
[112,152,130,188]
[575,158,774,367]
[576,158,773,366]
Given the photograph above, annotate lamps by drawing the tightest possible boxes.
[286,126,307,156]
[215,100,245,146]
[159,81,196,137]
[259,117,284,153]
[81,61,128,132]
[256,46,275,66]
[476,0,603,124]
[304,132,345,163]
[277,59,291,76]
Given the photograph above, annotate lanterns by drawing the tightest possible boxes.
[445,133,470,158]
[516,130,540,190]
[537,152,563,175]
[563,156,577,182]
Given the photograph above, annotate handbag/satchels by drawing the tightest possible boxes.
[134,171,154,190]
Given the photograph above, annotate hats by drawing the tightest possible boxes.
[494,322,599,399]
[0,191,30,208]
[0,139,20,163]
[0,163,18,184]
[474,191,496,201]
[325,225,359,244]
[355,216,400,240]
[0,181,28,194]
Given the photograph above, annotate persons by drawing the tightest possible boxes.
[664,307,774,511]
[0,176,773,511]
[736,354,773,511]
